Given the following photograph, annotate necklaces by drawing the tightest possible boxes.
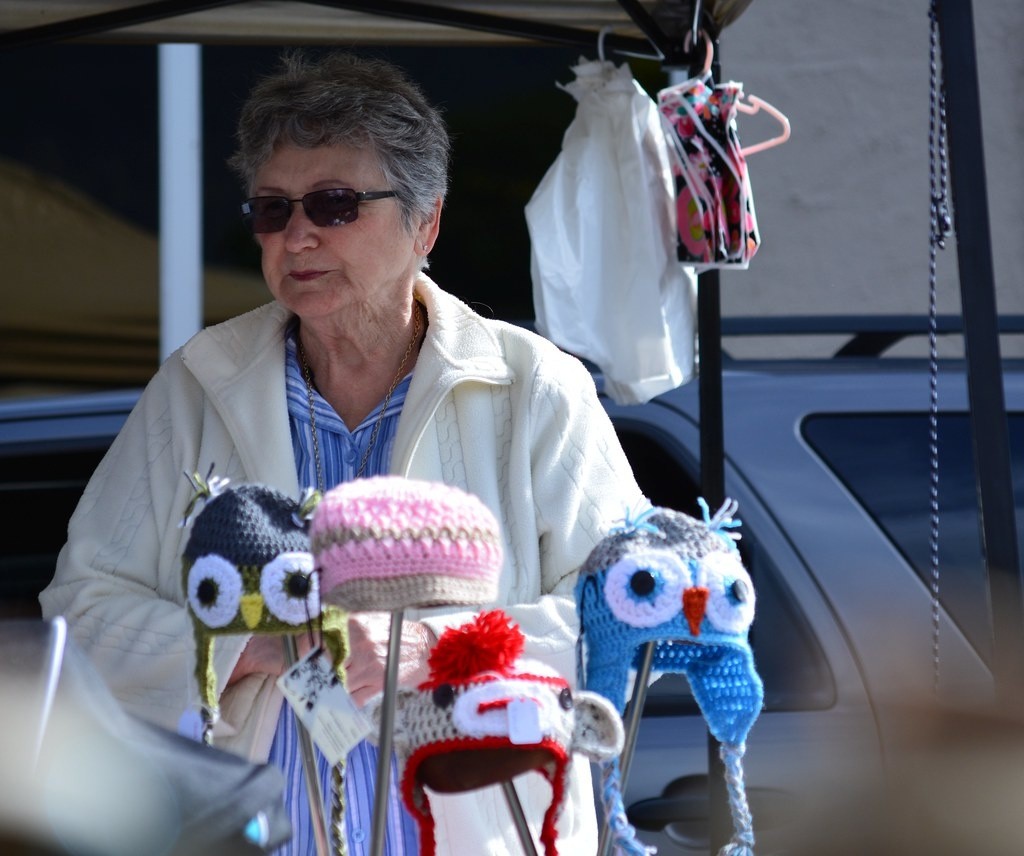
[293,304,421,492]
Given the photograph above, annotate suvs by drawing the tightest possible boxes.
[0,311,1023,856]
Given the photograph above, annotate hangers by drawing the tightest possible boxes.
[670,29,791,178]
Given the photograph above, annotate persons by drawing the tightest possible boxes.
[37,55,664,855]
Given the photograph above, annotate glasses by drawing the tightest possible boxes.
[239,189,400,235]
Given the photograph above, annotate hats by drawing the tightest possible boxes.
[575,497,765,856]
[357,612,625,856]
[179,463,348,746]
[313,477,502,613]
